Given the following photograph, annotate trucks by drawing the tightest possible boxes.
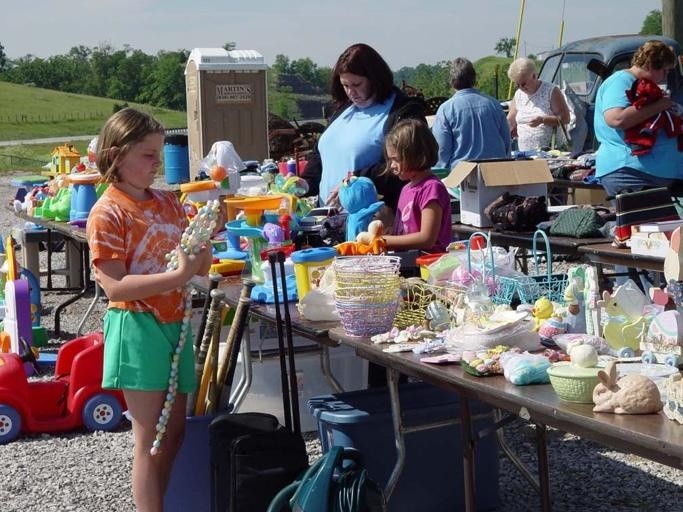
[502,33,683,156]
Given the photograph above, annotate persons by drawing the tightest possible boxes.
[593,40,681,205]
[86,107,210,512]
[356,118,452,256]
[296,43,427,234]
[503,57,571,154]
[430,56,514,169]
[415,88,423,99]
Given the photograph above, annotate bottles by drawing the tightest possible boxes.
[287,157,296,178]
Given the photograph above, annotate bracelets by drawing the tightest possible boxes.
[542,117,544,124]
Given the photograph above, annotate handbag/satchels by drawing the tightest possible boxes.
[485,192,547,230]
[541,139,573,152]
[550,205,608,237]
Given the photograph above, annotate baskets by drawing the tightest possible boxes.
[400,278,466,326]
[465,232,516,303]
[516,230,568,304]
[333,254,403,338]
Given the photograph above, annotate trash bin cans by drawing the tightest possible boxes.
[164,134,190,185]
